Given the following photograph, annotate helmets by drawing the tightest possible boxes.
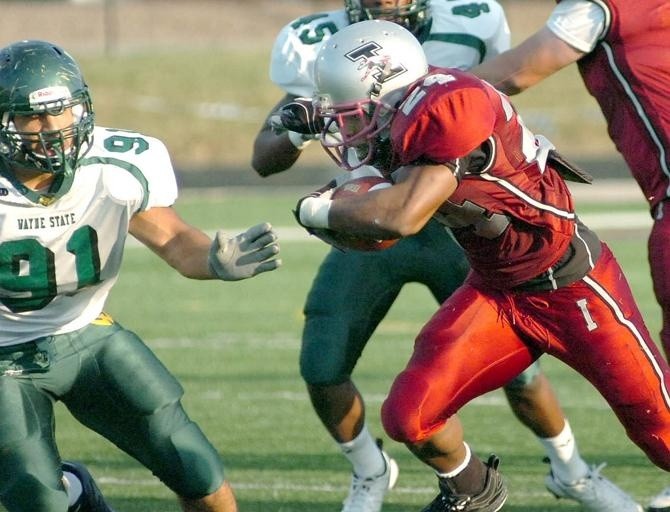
[313,19,429,171]
[0,40,93,177]
[343,0,433,34]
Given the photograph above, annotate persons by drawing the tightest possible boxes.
[0,39,287,511]
[293,17,670,512]
[252,1,649,512]
[465,0,670,376]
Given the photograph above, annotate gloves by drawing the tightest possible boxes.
[295,178,350,255]
[269,98,339,136]
[209,222,282,280]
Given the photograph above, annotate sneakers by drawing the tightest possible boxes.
[545,466,643,512]
[420,462,508,512]
[340,450,398,512]
[650,487,670,511]
[60,461,111,512]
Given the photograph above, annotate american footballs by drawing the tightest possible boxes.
[325,177,400,251]
